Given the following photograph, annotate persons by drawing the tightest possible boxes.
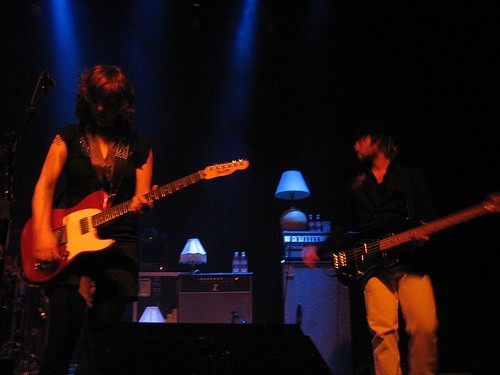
[300,124,439,375]
[29,63,158,375]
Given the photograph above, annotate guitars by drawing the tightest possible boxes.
[19,158,251,283]
[333,195,500,286]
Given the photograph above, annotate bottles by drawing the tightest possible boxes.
[315,214,321,231]
[232,252,240,272]
[240,252,248,273]
[308,214,314,231]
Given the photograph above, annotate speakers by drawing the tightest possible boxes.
[282,260,352,375]
[76,322,332,375]
[132,272,252,324]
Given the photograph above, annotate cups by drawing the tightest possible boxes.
[322,221,331,232]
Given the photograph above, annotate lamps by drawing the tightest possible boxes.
[179,238,208,274]
[273,170,314,231]
[138,306,166,323]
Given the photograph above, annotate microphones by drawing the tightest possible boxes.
[231,309,247,324]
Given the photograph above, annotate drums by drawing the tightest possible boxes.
[0,284,51,375]
